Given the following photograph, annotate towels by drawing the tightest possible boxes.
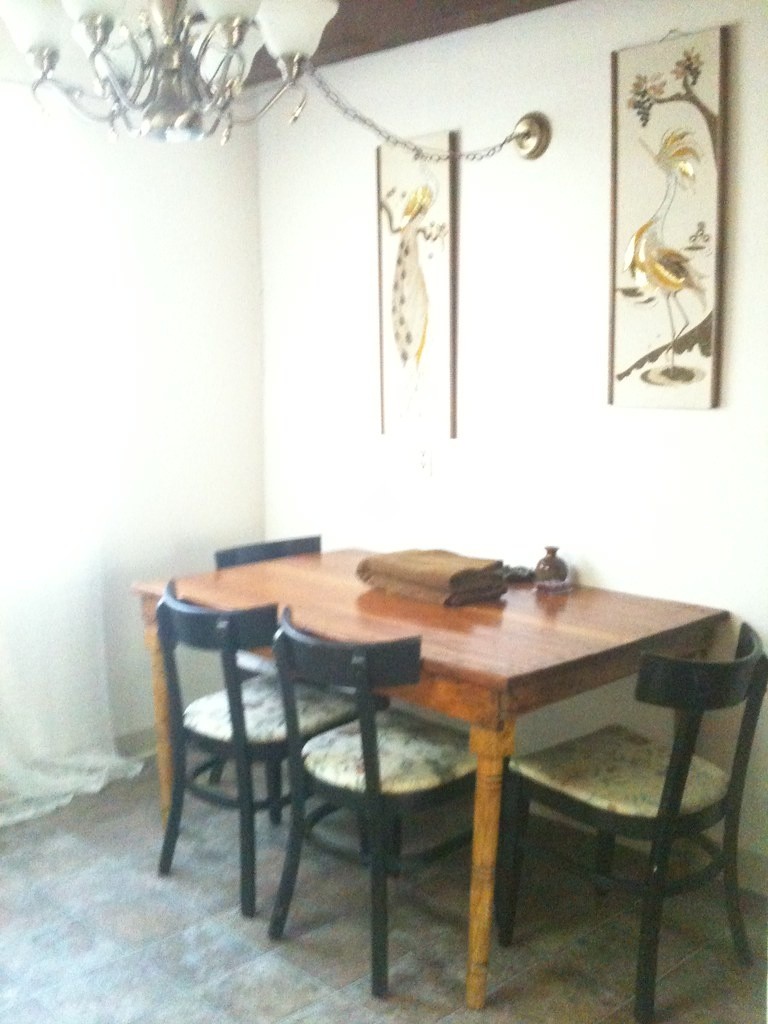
[356,549,511,608]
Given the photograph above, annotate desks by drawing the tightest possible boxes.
[129,547,728,1009]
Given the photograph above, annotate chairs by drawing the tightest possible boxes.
[268,605,478,996]
[207,534,325,824]
[505,619,768,1024]
[157,578,388,916]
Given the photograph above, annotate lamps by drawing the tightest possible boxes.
[0,1,341,145]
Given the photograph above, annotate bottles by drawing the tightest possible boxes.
[535,546,568,584]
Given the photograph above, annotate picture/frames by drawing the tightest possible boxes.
[376,127,456,437]
[611,24,720,411]
